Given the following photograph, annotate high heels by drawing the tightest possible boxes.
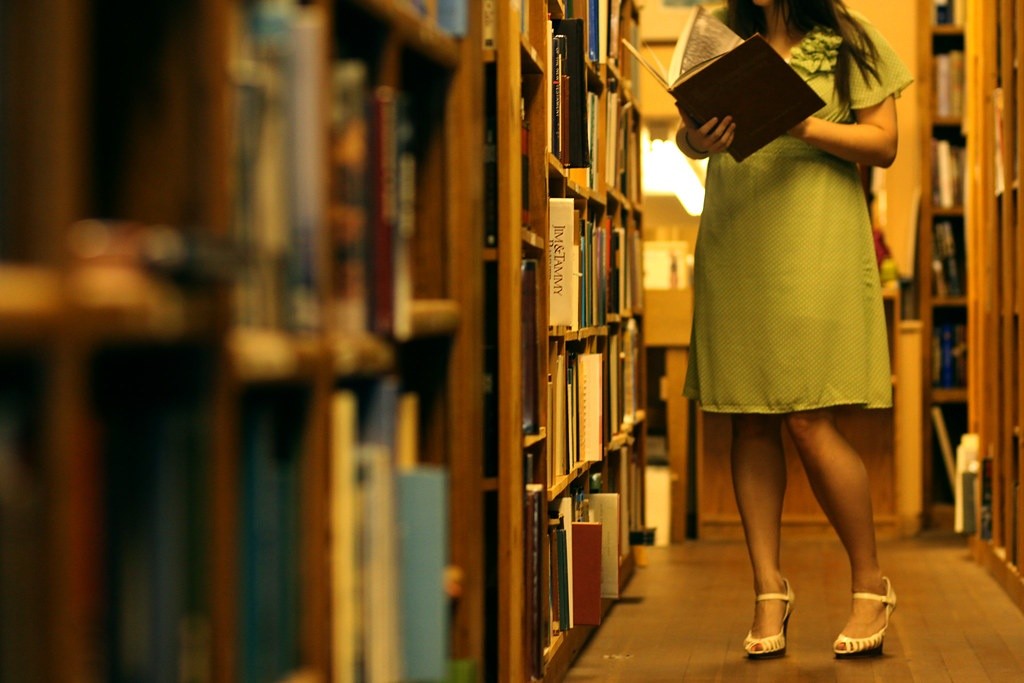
[742,577,796,660]
[832,577,896,659]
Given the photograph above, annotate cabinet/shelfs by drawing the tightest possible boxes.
[0,0,648,683]
[642,1,1018,547]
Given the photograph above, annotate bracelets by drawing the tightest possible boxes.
[685,130,708,156]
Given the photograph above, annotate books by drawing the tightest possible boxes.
[928,2,1023,581]
[4,0,648,683]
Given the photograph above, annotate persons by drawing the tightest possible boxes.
[672,0,915,660]
